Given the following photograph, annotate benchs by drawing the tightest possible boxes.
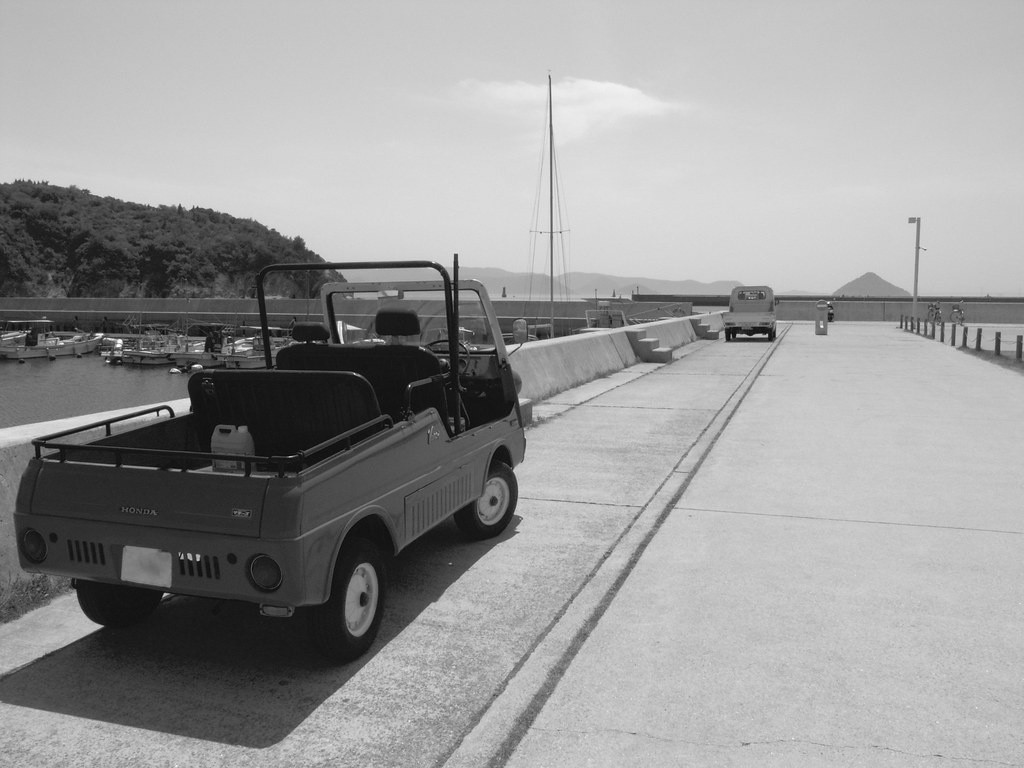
[187,306,453,454]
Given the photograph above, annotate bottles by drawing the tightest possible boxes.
[211,425,256,473]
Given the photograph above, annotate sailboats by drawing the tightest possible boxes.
[501,71,628,342]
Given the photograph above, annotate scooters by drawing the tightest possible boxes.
[827,304,833,322]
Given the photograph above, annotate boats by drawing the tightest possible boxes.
[103,314,476,370]
[0,319,104,359]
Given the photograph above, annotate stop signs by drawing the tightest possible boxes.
[816,300,828,311]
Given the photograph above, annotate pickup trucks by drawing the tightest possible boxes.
[12,254,531,662]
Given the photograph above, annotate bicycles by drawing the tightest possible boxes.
[951,300,965,325]
[928,304,942,325]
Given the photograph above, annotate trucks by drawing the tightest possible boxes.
[721,285,779,341]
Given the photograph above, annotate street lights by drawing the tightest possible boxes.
[907,216,921,330]
